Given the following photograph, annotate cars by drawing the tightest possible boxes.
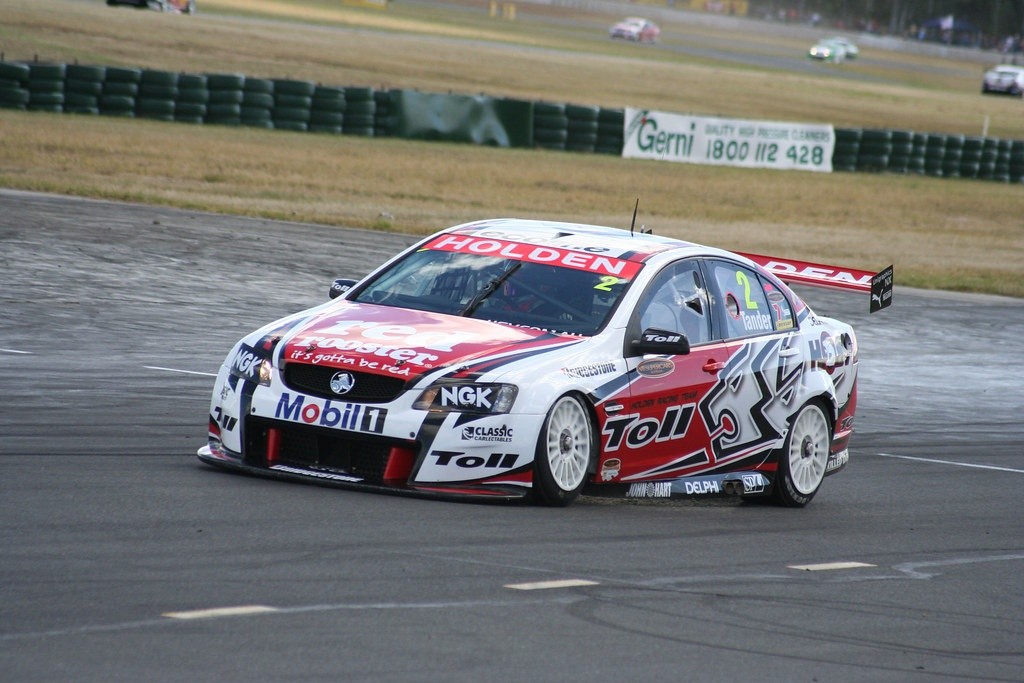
[197,198,859,506]
[610,16,659,43]
[808,37,858,59]
[982,64,1024,94]
